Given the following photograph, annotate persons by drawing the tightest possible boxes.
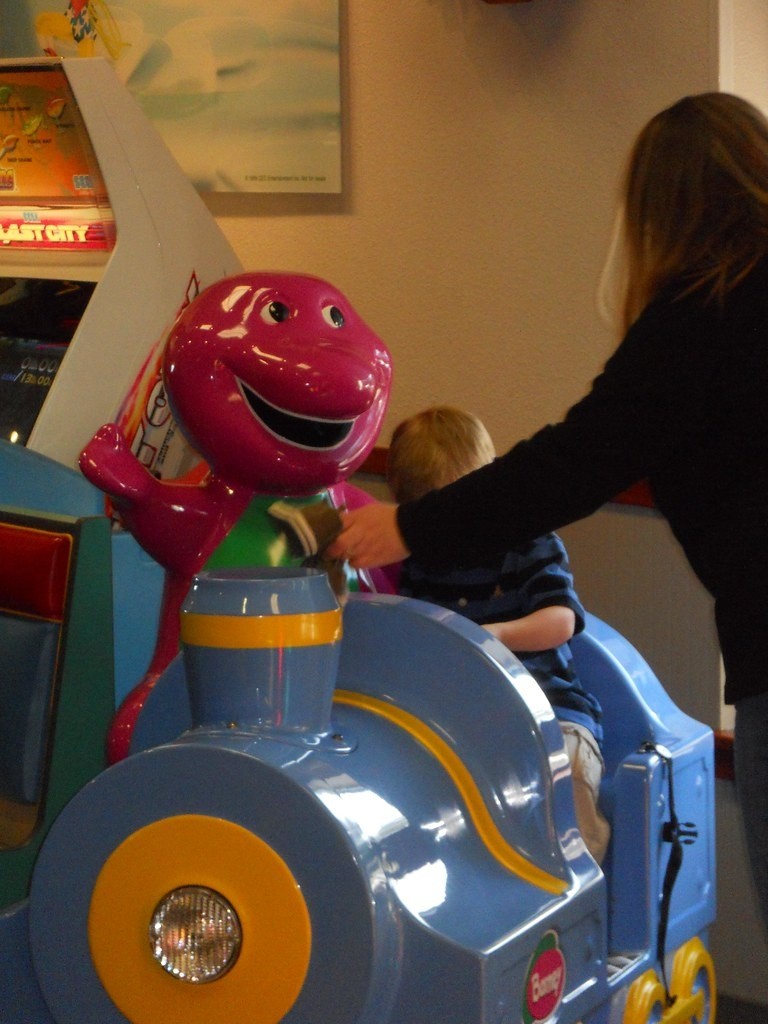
[388,407,609,861]
[323,93,768,934]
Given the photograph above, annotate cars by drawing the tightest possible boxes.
[1,269,718,1024]
